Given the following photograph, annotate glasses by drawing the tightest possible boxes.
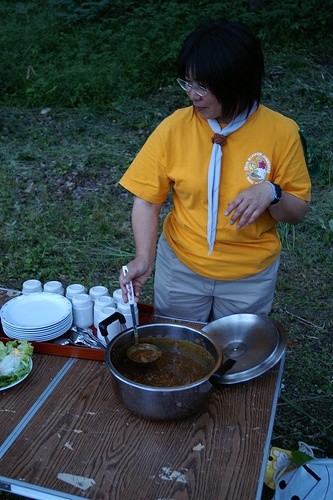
[176,77,211,96]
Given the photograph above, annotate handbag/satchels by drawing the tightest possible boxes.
[271,441,333,500]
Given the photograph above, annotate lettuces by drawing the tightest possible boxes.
[0,339,34,388]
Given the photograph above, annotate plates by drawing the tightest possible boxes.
[0,292,73,343]
[0,346,33,391]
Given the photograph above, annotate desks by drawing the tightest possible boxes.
[0,287,287,500]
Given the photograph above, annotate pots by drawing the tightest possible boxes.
[99,312,237,420]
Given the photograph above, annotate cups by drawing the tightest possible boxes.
[21,279,43,295]
[72,294,94,328]
[43,281,65,296]
[90,286,109,306]
[97,308,120,348]
[94,296,117,329]
[116,300,139,329]
[66,284,88,302]
[112,289,127,308]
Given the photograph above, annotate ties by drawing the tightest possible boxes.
[205,100,259,257]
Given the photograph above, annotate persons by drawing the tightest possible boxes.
[119,21,311,322]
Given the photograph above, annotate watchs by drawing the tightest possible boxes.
[268,182,282,205]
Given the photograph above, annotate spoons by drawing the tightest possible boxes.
[54,324,106,350]
